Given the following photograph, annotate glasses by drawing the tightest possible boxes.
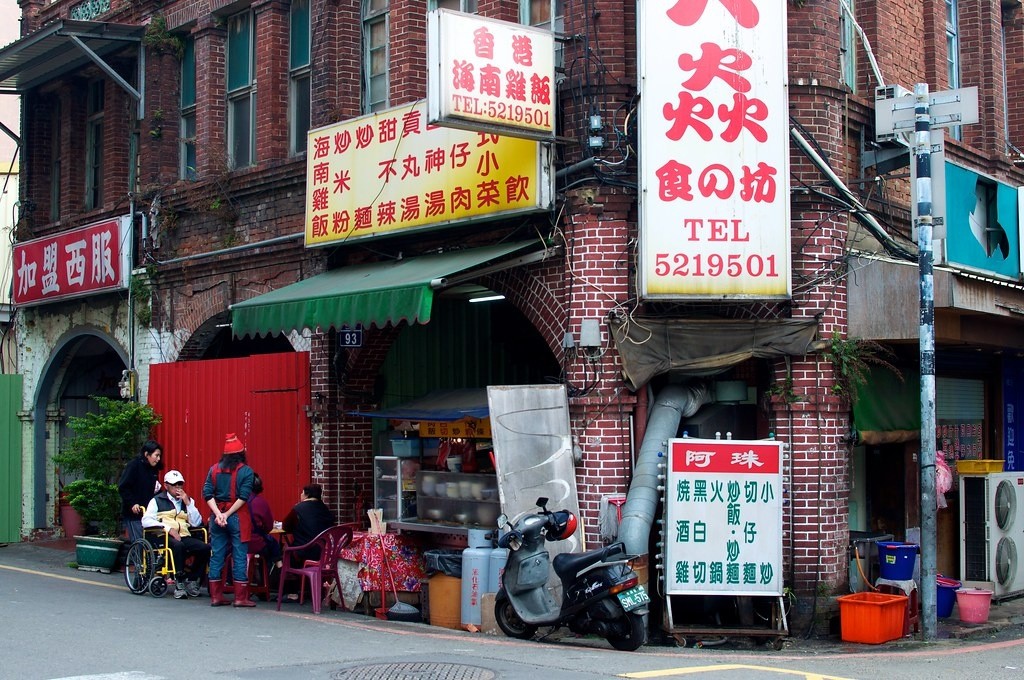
[165,481,183,487]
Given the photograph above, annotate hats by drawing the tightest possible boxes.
[163,470,186,486]
[224,433,244,455]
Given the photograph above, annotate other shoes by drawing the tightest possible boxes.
[276,594,300,603]
[133,582,150,592]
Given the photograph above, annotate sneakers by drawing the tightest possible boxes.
[185,581,200,597]
[173,582,187,599]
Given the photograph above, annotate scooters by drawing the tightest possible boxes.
[493,495,650,653]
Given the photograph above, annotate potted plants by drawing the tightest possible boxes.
[51,393,163,574]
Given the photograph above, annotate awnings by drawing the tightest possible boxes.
[228,236,550,342]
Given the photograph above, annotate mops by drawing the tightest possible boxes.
[597,492,626,542]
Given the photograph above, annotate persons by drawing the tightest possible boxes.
[247,472,283,601]
[202,434,257,607]
[282,484,334,606]
[141,470,210,600]
[117,441,165,595]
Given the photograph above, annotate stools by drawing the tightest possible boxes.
[222,552,270,602]
[874,577,920,638]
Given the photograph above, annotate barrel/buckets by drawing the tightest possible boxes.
[936,577,962,618]
[875,540,921,581]
[955,589,993,624]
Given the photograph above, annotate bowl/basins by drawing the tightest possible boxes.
[422,455,499,526]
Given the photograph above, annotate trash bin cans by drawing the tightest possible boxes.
[423,549,462,629]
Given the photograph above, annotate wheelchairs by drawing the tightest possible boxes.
[124,505,211,600]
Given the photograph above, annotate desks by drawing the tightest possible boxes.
[267,528,291,601]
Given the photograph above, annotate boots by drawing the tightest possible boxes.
[233,579,256,607]
[209,578,231,605]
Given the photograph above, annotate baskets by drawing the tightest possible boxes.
[956,460,1005,473]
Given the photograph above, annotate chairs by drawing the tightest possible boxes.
[277,523,354,615]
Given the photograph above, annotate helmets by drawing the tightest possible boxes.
[545,510,576,541]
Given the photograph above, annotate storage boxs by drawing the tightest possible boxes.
[836,592,909,644]
[390,437,440,458]
[955,459,1005,473]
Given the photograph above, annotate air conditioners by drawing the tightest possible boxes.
[875,84,914,146]
[959,471,1024,599]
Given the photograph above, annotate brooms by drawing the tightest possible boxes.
[374,513,421,622]
